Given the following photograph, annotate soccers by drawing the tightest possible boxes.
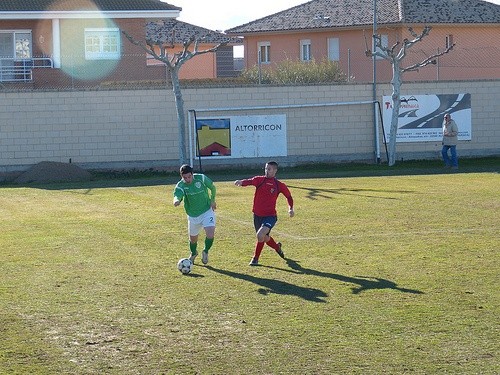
[177,258,193,274]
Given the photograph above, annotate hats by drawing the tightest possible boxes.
[444,113,451,118]
[179,165,193,173]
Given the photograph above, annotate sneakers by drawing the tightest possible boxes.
[249,257,258,265]
[189,251,198,260]
[276,242,284,259]
[202,250,208,263]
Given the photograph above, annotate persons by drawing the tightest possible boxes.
[234,161,295,266]
[442,114,458,170]
[173,164,216,265]
